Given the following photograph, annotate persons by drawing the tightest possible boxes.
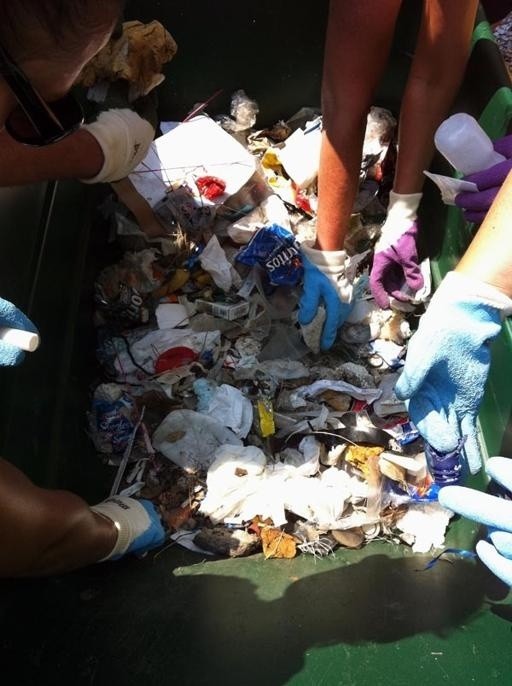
[1,2,169,568]
[301,0,512,591]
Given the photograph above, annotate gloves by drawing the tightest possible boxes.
[79,107,155,185]
[0,297,41,368]
[298,238,356,354]
[437,455,511,588]
[453,137,511,225]
[89,494,167,564]
[393,270,512,476]
[369,189,424,305]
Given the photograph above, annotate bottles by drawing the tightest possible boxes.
[434,114,507,176]
